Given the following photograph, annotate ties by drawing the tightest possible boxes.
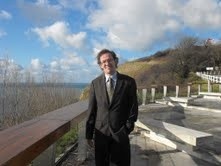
[107,77,114,105]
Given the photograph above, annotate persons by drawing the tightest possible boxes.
[86,48,138,166]
